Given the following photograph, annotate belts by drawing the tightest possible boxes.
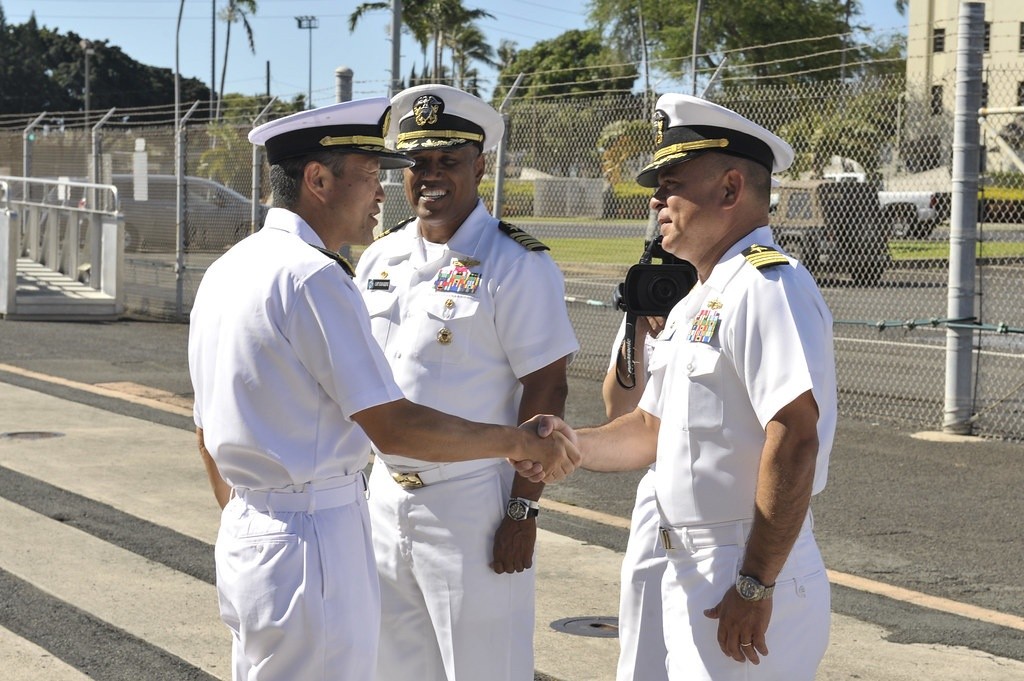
[234,474,368,512]
[383,458,503,491]
[659,511,812,550]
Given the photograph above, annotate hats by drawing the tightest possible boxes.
[384,84,506,157]
[247,97,417,171]
[636,92,794,189]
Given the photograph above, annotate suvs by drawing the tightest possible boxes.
[771,178,889,287]
[37,172,270,255]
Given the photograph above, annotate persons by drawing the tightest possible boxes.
[353,84,581,680]
[185,96,580,680]
[505,92,836,681]
[603,255,667,681]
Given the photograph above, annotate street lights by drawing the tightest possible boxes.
[79,39,96,145]
[294,15,320,111]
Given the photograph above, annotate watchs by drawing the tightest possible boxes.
[506,497,539,521]
[735,570,775,601]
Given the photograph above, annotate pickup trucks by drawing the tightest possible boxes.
[819,170,952,238]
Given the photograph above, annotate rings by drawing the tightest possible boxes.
[739,642,752,647]
